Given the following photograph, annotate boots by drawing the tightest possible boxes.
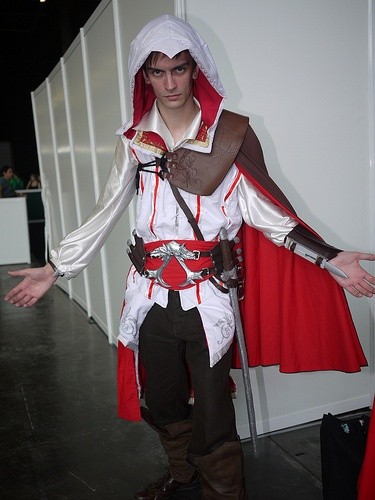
[134,405,247,500]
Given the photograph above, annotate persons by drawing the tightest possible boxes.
[3,14,375,499]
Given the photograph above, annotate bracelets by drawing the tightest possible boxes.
[47,260,64,277]
